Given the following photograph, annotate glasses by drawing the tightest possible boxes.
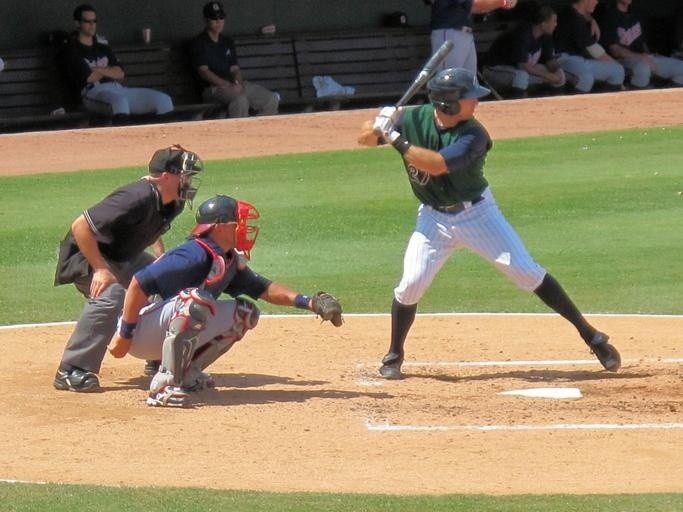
[210,13,225,21]
[80,18,98,24]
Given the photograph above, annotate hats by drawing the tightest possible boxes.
[386,11,409,28]
[203,1,227,19]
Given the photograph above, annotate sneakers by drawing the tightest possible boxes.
[144,358,161,376]
[185,372,216,391]
[585,332,620,372]
[53,367,100,393]
[377,353,404,380]
[147,386,192,407]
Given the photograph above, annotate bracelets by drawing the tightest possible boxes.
[390,135,411,155]
[120,319,137,339]
[294,294,311,310]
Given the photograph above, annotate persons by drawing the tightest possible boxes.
[357,68,620,380]
[106,195,342,408]
[186,2,279,119]
[544,0,625,94]
[51,144,203,393]
[49,5,175,127]
[598,0,682,90]
[483,6,567,99]
[430,0,517,73]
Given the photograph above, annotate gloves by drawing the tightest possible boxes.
[376,106,404,126]
[374,116,410,157]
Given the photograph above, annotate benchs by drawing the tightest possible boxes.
[0,22,646,135]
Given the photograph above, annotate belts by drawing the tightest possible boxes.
[81,79,119,95]
[428,196,483,216]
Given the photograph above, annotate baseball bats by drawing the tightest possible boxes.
[373,41,454,137]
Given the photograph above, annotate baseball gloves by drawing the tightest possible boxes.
[312,291,342,328]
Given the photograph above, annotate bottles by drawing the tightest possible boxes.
[398,8,407,27]
[141,28,152,46]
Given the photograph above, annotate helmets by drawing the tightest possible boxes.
[426,67,491,115]
[190,196,259,261]
[148,145,205,211]
